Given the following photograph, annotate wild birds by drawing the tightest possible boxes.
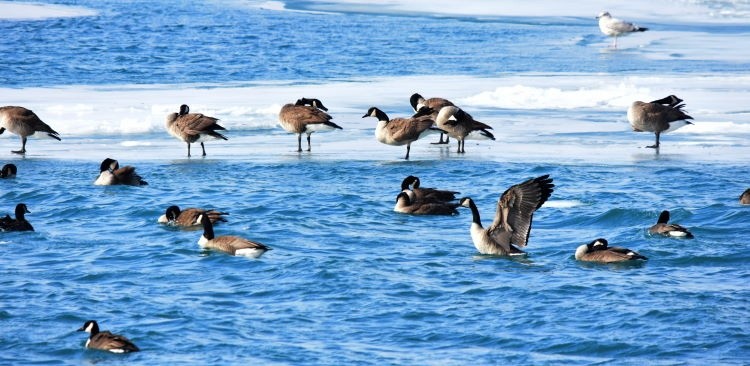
[394,176,465,217]
[158,205,229,228]
[77,320,140,354]
[455,174,555,256]
[575,238,648,263]
[739,188,750,205]
[0,106,62,178]
[166,105,229,158]
[192,212,273,255]
[649,209,694,239]
[278,97,343,152]
[627,94,695,148]
[363,107,439,160]
[596,11,649,49]
[0,203,35,232]
[94,158,148,186]
[410,93,496,153]
